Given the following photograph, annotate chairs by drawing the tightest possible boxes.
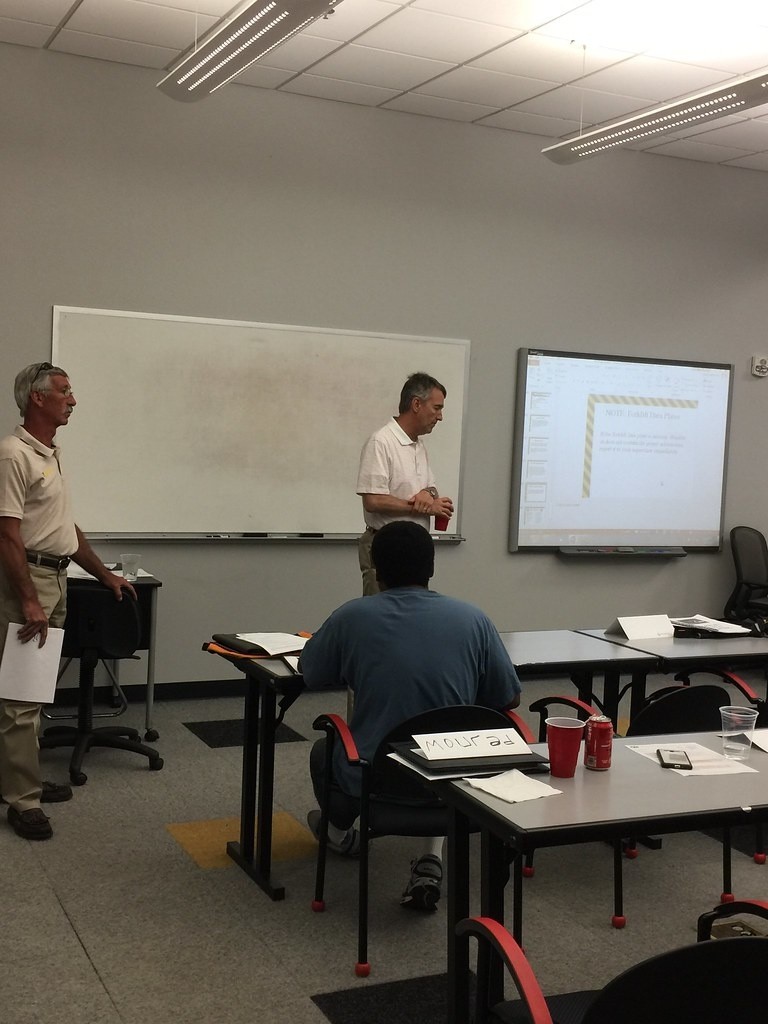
[724,526,768,617]
[39,588,163,785]
[313,666,768,1024]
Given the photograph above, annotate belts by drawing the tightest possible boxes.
[27,553,71,570]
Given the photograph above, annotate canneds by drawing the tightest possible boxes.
[584,715,612,770]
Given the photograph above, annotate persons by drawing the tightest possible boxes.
[356,373,454,598]
[0,362,137,841]
[298,520,523,915]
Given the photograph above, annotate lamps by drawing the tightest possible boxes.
[542,72,768,165]
[155,0,343,103]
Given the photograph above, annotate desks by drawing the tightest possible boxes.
[580,628,768,850]
[399,729,768,1024]
[205,628,661,900]
[64,563,162,742]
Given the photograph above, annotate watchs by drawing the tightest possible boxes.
[423,488,436,496]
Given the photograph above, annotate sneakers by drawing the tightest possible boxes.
[400,854,443,913]
[308,811,361,857]
[0,781,73,803]
[7,805,53,840]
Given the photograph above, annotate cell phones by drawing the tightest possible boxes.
[657,749,692,770]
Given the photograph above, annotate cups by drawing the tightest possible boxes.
[544,717,586,777]
[120,554,141,581]
[719,706,759,759]
[435,515,448,531]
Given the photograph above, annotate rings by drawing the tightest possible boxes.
[424,508,426,510]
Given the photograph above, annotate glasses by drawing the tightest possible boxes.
[31,362,53,392]
[29,385,73,399]
